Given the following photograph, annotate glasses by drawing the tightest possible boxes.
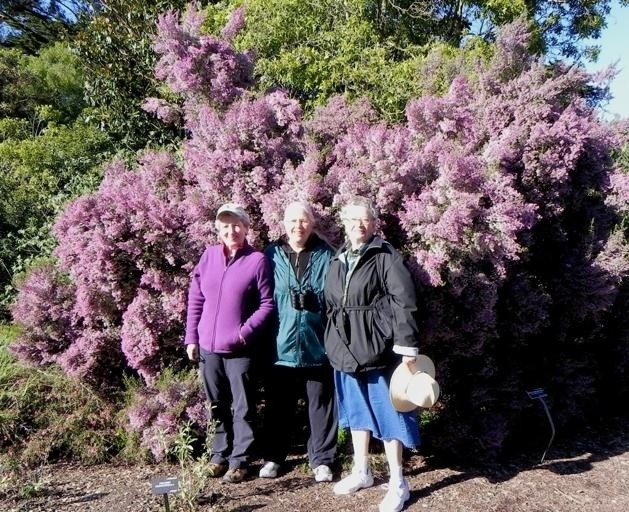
[349,217,371,224]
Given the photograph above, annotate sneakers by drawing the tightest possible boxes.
[378,478,410,512]
[222,465,248,483]
[259,460,281,478]
[333,466,375,495]
[202,462,226,477]
[312,465,333,482]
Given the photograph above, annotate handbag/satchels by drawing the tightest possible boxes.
[372,253,394,339]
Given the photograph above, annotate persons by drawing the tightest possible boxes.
[257,201,341,485]
[319,196,423,510]
[183,200,275,484]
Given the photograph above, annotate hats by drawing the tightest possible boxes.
[216,203,250,225]
[389,355,440,413]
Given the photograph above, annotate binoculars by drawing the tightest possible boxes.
[290,289,313,308]
